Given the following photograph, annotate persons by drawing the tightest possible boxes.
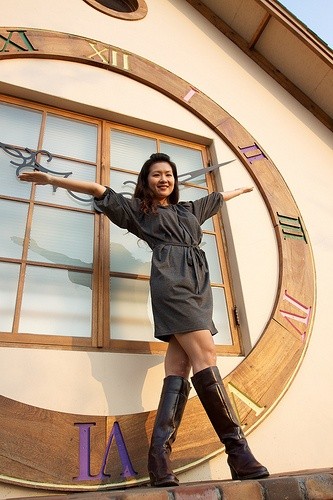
[18,153,270,486]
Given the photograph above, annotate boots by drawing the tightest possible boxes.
[147,374,192,487]
[190,366,270,480]
